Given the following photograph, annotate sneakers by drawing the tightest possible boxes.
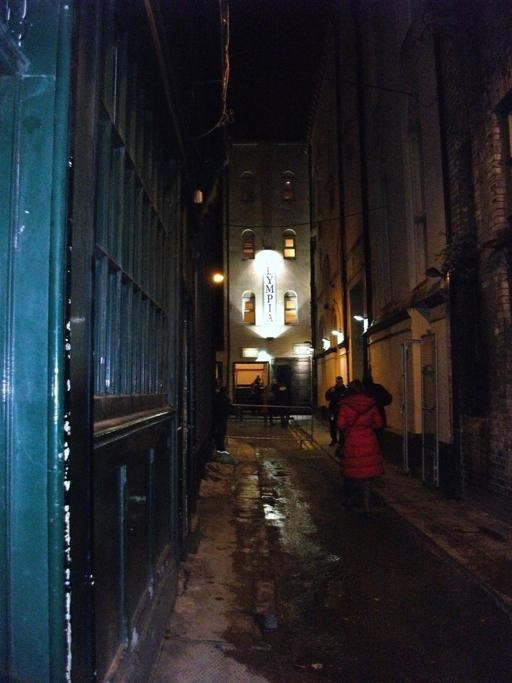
[344,510,372,521]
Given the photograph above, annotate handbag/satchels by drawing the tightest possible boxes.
[334,444,344,457]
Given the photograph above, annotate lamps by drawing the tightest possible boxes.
[351,312,369,320]
[422,265,450,279]
[319,336,331,342]
[303,339,313,345]
[263,335,276,341]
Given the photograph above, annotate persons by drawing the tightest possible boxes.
[361,374,392,445]
[326,377,350,446]
[214,387,233,455]
[251,374,292,427]
[336,380,382,517]
